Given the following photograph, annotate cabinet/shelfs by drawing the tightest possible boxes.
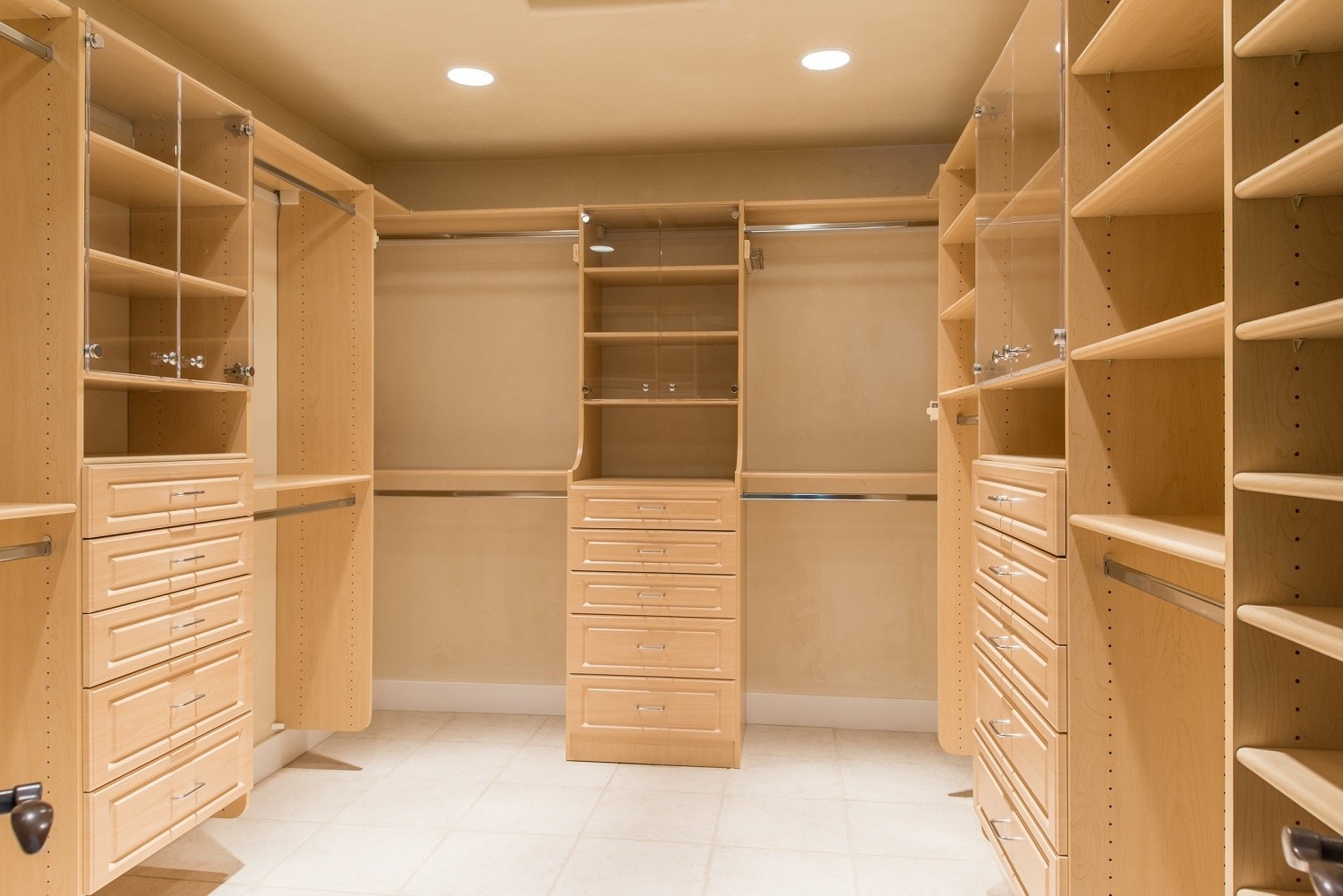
[0,0,1343,896]
